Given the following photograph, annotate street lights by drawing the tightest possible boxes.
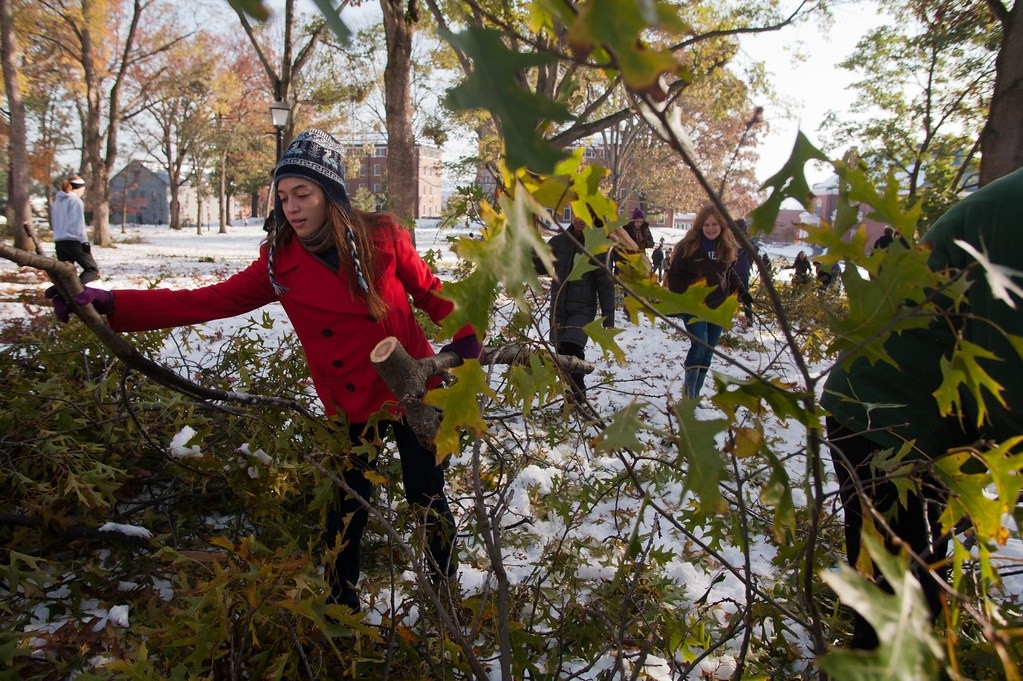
[267,95,293,165]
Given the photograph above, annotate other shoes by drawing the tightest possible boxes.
[433,570,459,613]
[560,393,586,412]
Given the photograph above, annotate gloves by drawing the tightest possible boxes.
[440,334,484,366]
[741,289,752,303]
[82,242,91,253]
[45,284,114,324]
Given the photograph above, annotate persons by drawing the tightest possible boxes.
[662,247,674,286]
[651,244,665,282]
[615,207,652,322]
[783,250,812,297]
[819,167,1023,651]
[660,237,665,244]
[46,175,100,298]
[813,257,841,296]
[51,128,488,622]
[532,203,615,409]
[668,205,754,409]
[730,219,758,326]
[758,253,772,283]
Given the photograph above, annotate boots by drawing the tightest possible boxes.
[695,372,707,409]
[685,368,699,400]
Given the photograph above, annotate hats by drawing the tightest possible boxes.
[632,208,644,219]
[268,128,370,296]
